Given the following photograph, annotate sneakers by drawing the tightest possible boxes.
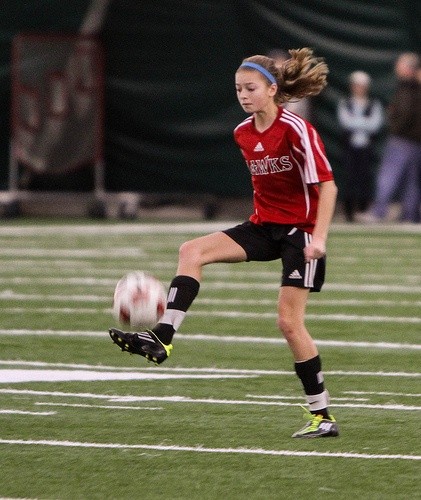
[291,403,338,438]
[107,325,173,365]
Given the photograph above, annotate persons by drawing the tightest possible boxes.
[337,71,383,222]
[353,52,421,223]
[108,48,339,440]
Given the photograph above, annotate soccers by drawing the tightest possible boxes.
[113,271,169,332]
